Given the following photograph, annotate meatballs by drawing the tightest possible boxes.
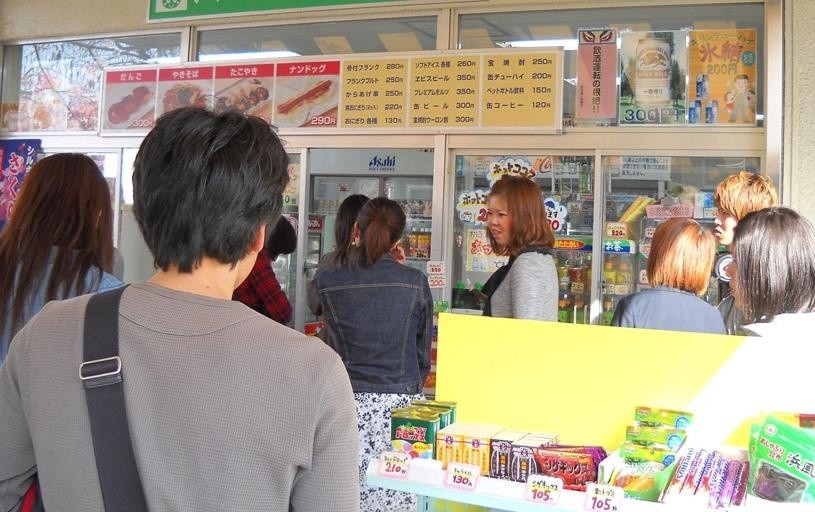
[108,86,150,124]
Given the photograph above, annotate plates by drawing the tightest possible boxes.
[103,76,339,130]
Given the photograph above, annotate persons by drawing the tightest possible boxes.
[0,152,125,510]
[0,104,361,512]
[610,217,728,336]
[713,172,782,336]
[483,174,559,322]
[230,213,299,326]
[308,193,370,317]
[315,196,434,512]
[726,206,815,339]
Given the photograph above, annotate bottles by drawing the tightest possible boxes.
[407,227,430,258]
[554,254,632,326]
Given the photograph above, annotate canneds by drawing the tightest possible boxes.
[686,72,718,125]
[558,264,590,292]
[390,400,457,460]
[632,36,672,105]
